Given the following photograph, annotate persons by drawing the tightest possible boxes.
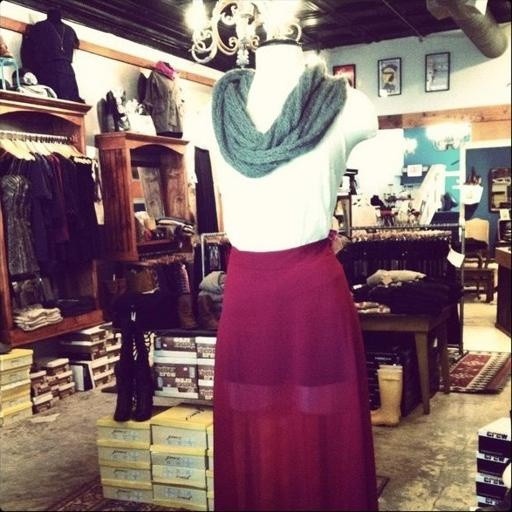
[21,8,85,103]
[142,61,186,138]
[201,39,378,510]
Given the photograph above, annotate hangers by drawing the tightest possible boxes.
[197,223,464,247]
[0,129,93,174]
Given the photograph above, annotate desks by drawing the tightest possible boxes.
[464,266,497,303]
[492,248,512,338]
[357,308,452,417]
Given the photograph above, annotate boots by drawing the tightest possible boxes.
[176,294,197,329]
[196,293,221,330]
[132,359,154,423]
[113,361,134,421]
[370,365,404,427]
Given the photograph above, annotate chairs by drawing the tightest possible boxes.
[464,217,491,269]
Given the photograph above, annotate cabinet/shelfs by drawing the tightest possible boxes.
[96,131,195,262]
[488,166,512,246]
[1,88,106,352]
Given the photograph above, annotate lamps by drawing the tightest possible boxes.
[177,1,323,68]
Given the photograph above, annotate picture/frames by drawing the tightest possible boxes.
[333,63,357,90]
[377,55,402,98]
[424,51,451,93]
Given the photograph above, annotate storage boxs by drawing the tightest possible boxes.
[97,334,217,512]
[0,316,124,426]
[476,417,512,512]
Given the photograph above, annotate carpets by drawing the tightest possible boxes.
[43,459,390,511]
[440,348,511,396]
